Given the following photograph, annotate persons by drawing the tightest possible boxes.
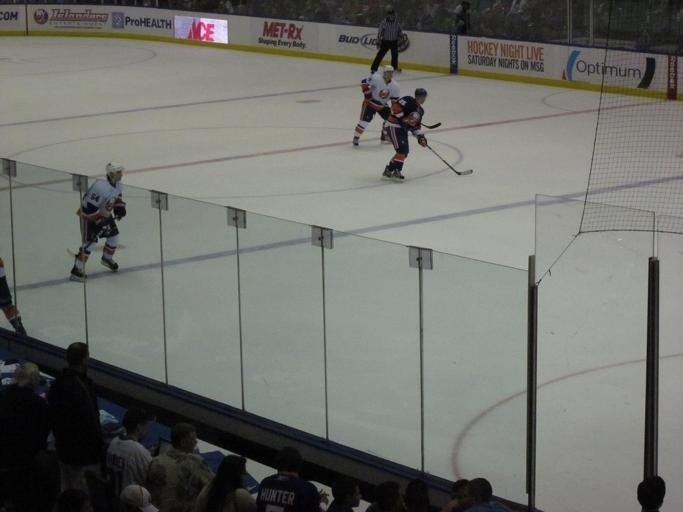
[68,161,126,282]
[351,64,400,147]
[1,361,51,512]
[105,409,152,500]
[195,447,512,511]
[635,475,665,511]
[121,2,680,54]
[118,484,158,511]
[44,342,105,512]
[380,87,427,183]
[55,488,94,512]
[369,9,401,72]
[145,420,214,511]
[0,259,28,336]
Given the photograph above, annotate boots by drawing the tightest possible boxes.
[102,255,118,269]
[353,130,406,180]
[71,264,87,279]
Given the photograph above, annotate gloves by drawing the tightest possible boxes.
[96,217,110,237]
[364,90,373,102]
[114,201,126,217]
[417,135,427,147]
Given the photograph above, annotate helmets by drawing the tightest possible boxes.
[105,163,122,174]
[415,88,427,96]
[384,65,394,72]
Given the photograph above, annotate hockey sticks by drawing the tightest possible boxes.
[425,143,473,175]
[420,122,441,128]
[67,216,117,256]
